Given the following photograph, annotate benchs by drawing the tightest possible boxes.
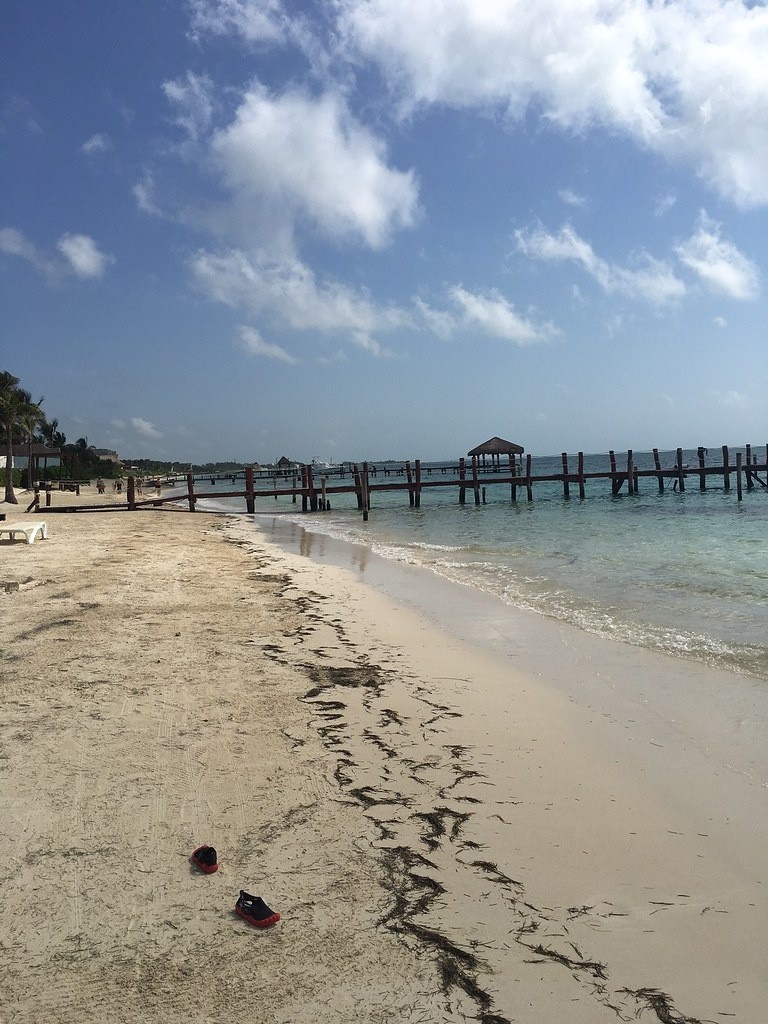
[0,522,47,544]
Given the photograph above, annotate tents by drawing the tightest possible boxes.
[466,437,523,469]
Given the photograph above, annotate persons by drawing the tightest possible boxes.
[133,476,144,495]
[115,474,124,493]
[155,479,161,486]
[96,476,106,494]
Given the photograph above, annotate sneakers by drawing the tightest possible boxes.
[236,890,280,927]
[192,845,218,873]
